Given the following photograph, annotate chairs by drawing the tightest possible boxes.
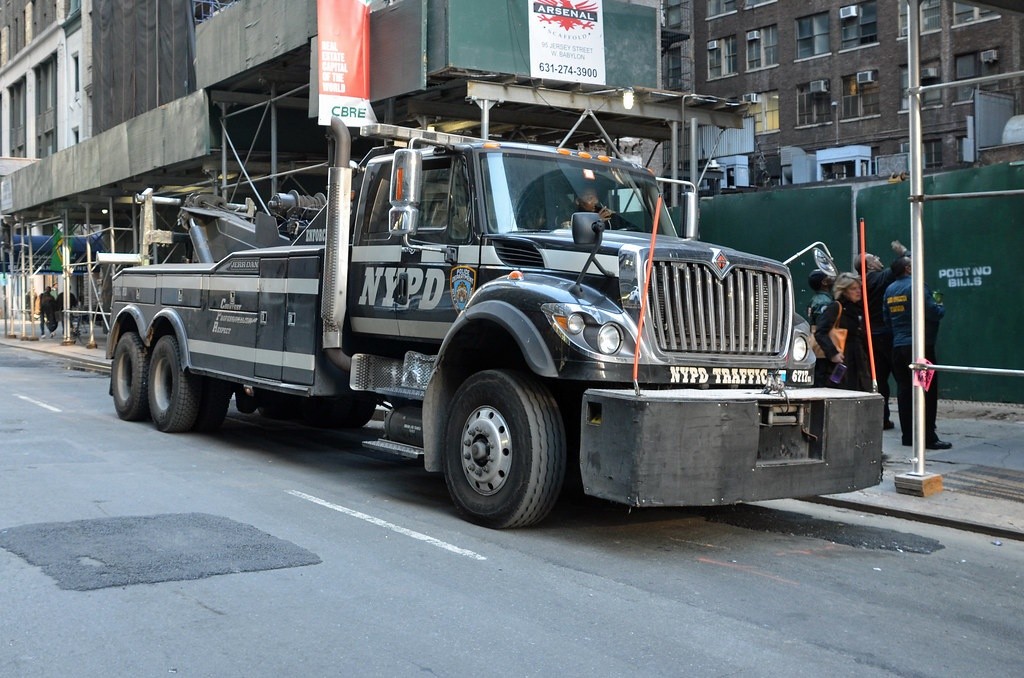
[427,192,469,230]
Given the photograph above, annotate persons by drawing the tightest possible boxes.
[39,283,87,339]
[574,186,641,231]
[808,241,952,458]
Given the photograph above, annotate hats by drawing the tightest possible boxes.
[808,269,828,290]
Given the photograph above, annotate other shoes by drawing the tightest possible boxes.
[51,332,55,338]
[884,421,894,430]
[41,334,46,339]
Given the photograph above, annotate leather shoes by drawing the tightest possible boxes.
[926,440,952,449]
[902,439,912,446]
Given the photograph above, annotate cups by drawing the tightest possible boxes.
[933,292,943,306]
[830,363,847,384]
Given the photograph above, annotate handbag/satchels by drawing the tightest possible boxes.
[810,301,848,359]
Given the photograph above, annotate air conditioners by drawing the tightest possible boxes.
[742,93,759,104]
[980,49,999,63]
[840,6,857,19]
[708,41,717,49]
[810,80,828,94]
[920,68,939,79]
[856,71,874,83]
[746,30,759,41]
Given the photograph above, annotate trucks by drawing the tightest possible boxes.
[106,118,885,530]
